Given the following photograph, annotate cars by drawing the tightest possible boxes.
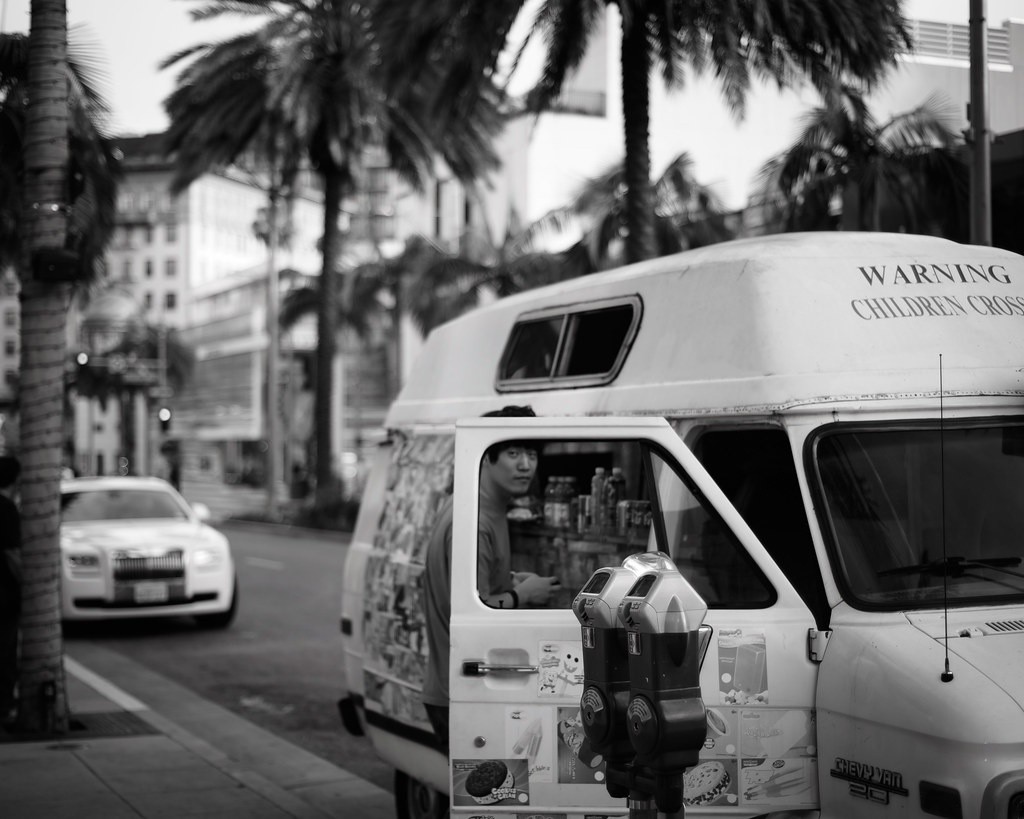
[57,470,238,632]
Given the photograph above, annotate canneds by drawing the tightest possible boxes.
[616,499,651,539]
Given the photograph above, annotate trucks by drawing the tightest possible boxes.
[331,228,1024,819]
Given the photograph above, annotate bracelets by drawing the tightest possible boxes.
[505,590,519,609]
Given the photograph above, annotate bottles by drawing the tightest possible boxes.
[593,466,626,534]
[540,475,576,530]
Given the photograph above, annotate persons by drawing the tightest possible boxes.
[60,462,75,481]
[111,447,135,475]
[423,406,561,757]
[153,444,178,483]
[291,460,314,510]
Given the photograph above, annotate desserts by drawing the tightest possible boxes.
[464,760,516,805]
[684,761,732,806]
